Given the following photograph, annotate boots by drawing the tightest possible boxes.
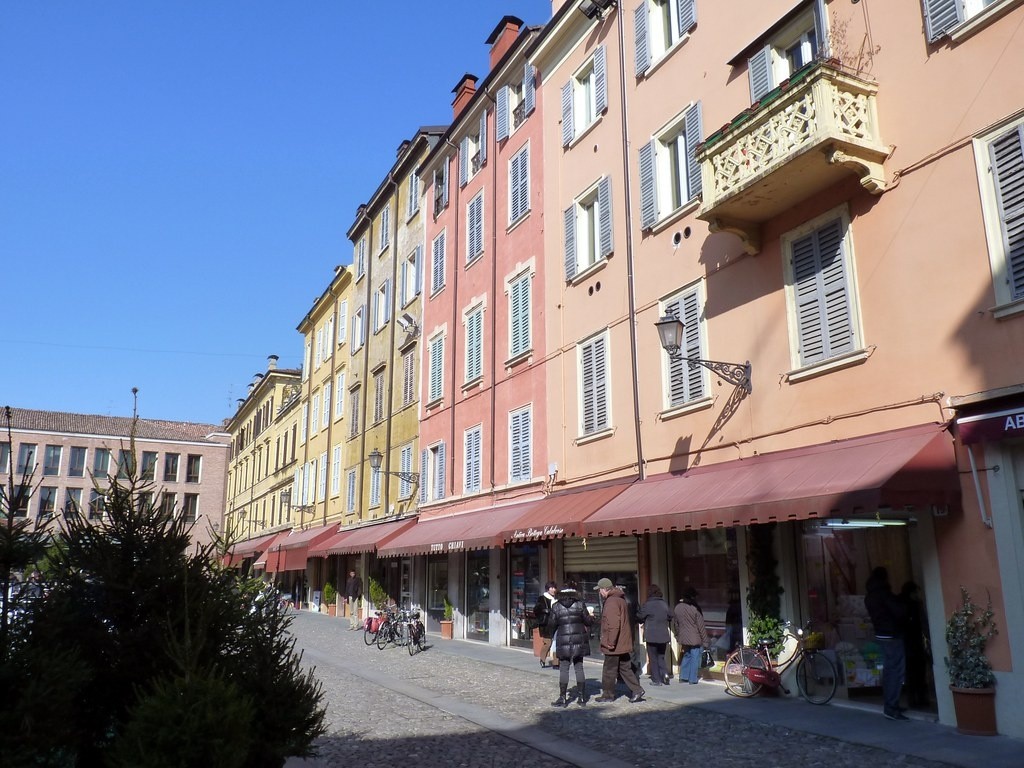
[551,695,566,708]
[577,695,586,707]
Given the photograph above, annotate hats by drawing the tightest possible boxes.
[592,577,613,590]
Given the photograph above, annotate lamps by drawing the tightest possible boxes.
[397,317,413,336]
[280,489,316,515]
[213,521,226,533]
[591,0,618,10]
[578,0,604,22]
[654,307,753,395]
[368,447,419,487]
[238,508,267,528]
[402,312,418,328]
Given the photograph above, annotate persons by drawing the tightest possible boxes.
[726,597,743,663]
[344,570,363,632]
[864,566,931,723]
[673,587,712,685]
[292,574,309,611]
[635,585,675,686]
[533,579,590,671]
[548,581,598,708]
[593,578,645,703]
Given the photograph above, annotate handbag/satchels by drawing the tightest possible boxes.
[701,650,715,669]
[370,618,380,632]
[363,617,372,632]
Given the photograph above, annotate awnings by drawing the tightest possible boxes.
[499,482,632,544]
[581,426,963,540]
[222,516,419,572]
[957,401,1024,446]
[375,497,542,557]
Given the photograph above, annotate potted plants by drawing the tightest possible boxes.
[439,598,456,640]
[323,581,338,616]
[943,583,999,736]
[357,598,363,619]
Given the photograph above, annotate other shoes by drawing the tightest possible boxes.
[664,674,670,685]
[553,665,560,670]
[883,703,909,722]
[595,696,615,702]
[629,689,645,702]
[348,627,359,631]
[678,678,689,683]
[650,681,662,686]
[540,660,545,668]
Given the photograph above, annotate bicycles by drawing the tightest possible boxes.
[364,603,426,656]
[263,599,293,616]
[723,619,837,706]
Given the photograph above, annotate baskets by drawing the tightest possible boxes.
[802,631,826,655]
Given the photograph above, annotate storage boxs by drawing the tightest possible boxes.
[837,623,871,641]
